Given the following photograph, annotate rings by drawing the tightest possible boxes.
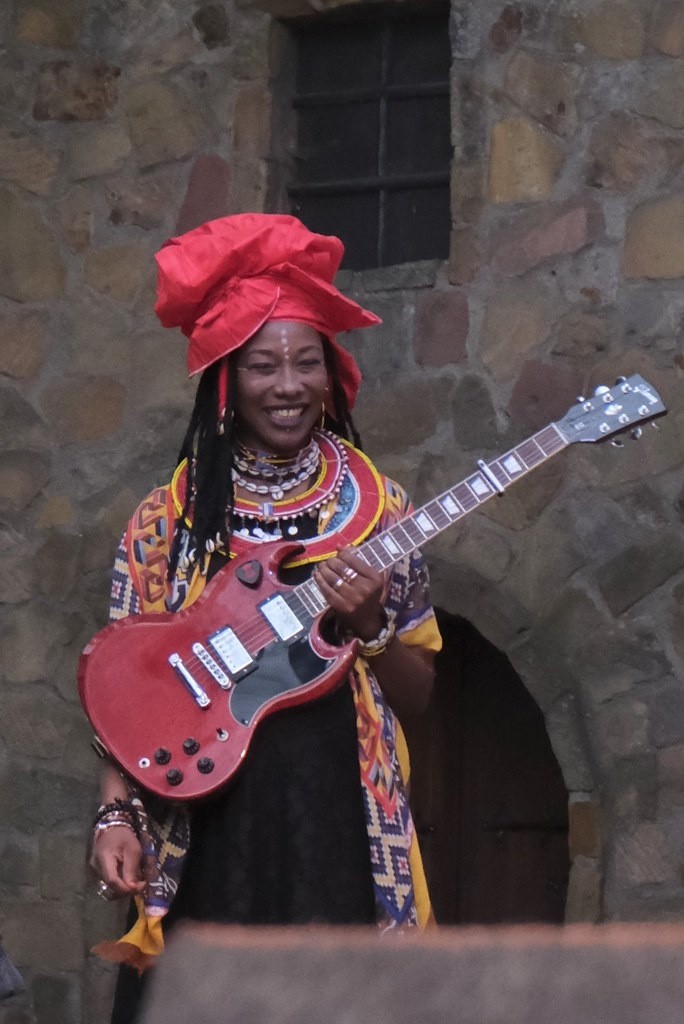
[333,578,343,591]
[340,567,358,582]
[96,879,114,902]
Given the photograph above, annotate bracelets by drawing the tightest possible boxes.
[93,797,139,842]
[343,606,398,658]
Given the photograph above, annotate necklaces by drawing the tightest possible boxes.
[230,435,322,501]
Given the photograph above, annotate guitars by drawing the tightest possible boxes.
[78,374,670,796]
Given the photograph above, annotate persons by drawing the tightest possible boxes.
[90,212,443,971]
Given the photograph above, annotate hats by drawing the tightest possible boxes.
[155,212,383,435]
